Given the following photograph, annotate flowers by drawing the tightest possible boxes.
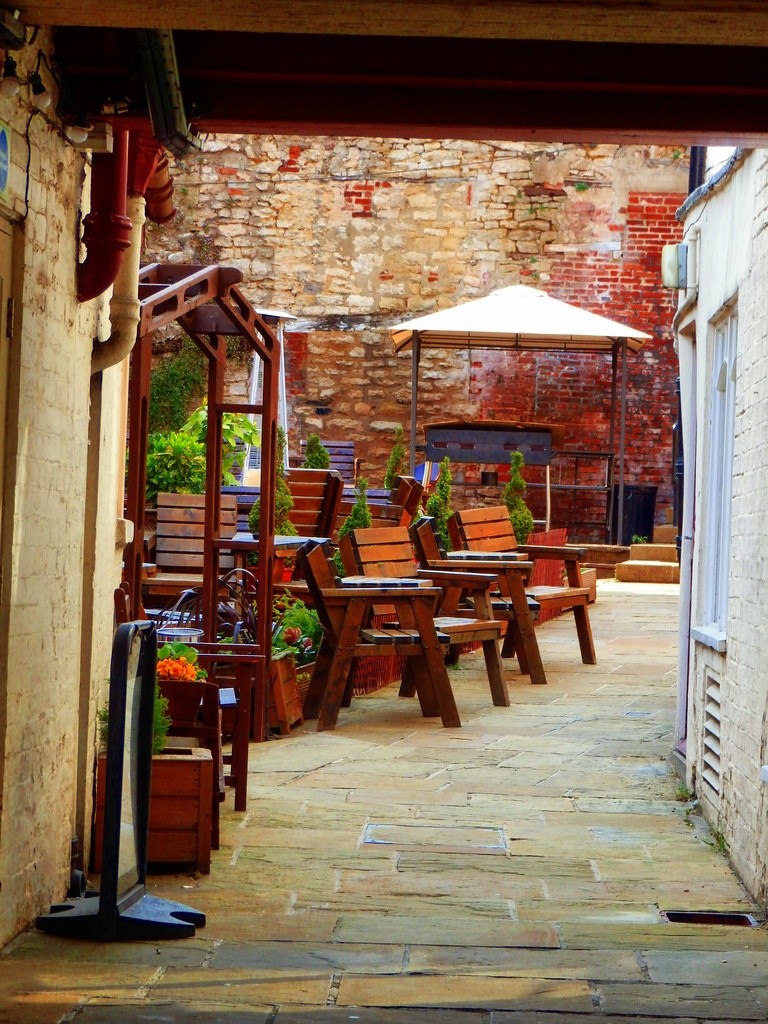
[155,641,210,682]
[269,586,323,665]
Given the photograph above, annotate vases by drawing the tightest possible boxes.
[158,679,208,724]
[294,660,316,708]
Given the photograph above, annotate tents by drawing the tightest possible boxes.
[387,285,654,547]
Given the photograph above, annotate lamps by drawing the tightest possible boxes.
[660,243,687,288]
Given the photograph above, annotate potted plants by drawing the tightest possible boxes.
[97,675,211,875]
[562,568,596,611]
[152,568,305,736]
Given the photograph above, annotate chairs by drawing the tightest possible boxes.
[143,470,596,849]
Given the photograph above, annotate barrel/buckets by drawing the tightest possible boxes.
[606,481,659,546]
[157,628,204,642]
